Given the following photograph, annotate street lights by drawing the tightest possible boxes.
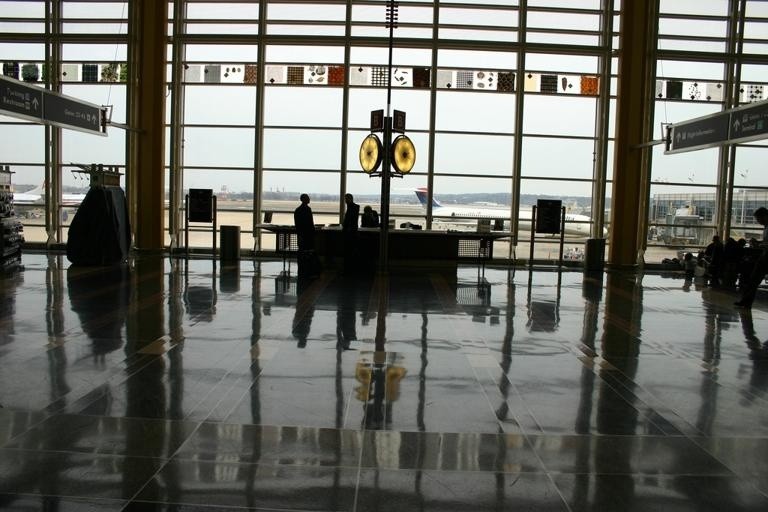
[688,176,697,208]
[655,176,666,222]
[740,172,747,224]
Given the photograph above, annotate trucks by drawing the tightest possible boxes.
[662,213,703,249]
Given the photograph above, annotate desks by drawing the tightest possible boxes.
[447,229,516,257]
[254,224,325,259]
[320,225,459,281]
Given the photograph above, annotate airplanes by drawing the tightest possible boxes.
[62,194,86,206]
[13,174,53,220]
[412,187,608,238]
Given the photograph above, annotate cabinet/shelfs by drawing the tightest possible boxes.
[0,171,23,270]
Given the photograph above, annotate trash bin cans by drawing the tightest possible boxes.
[219,225,241,260]
[584,238,605,270]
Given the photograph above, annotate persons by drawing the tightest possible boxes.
[678,234,763,290]
[359,206,379,228]
[293,193,316,246]
[735,207,768,308]
[343,192,358,227]
